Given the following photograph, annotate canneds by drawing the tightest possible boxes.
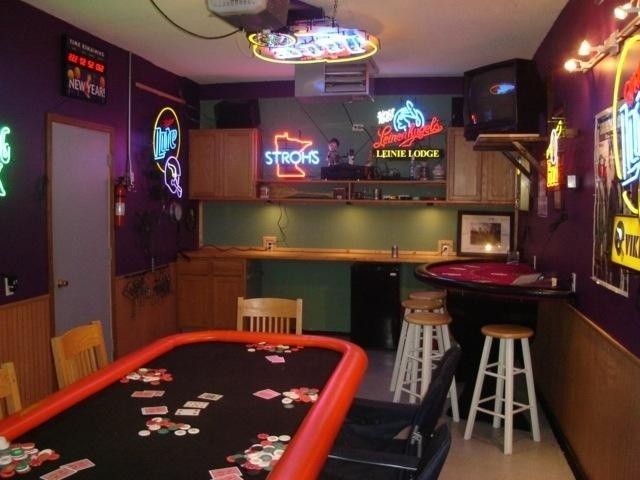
[373,187,382,200]
[392,246,398,258]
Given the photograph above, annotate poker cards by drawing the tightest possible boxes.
[174,392,224,416]
[39,458,97,480]
[208,466,244,480]
[131,390,166,398]
[252,388,281,399]
[141,405,169,415]
[265,355,285,364]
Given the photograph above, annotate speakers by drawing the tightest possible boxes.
[214,98,262,129]
[452,97,464,127]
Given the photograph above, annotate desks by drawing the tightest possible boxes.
[0,330,370,479]
[414,260,572,432]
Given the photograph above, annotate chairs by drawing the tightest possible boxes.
[319,345,461,479]
[1,318,108,424]
[235,298,303,336]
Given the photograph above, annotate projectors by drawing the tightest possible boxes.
[206,0,289,31]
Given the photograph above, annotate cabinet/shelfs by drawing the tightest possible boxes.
[259,178,446,206]
[188,128,263,202]
[176,258,262,332]
[446,127,520,204]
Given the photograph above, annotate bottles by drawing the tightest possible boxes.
[392,245,398,258]
[373,187,380,200]
[409,154,416,180]
[420,161,445,180]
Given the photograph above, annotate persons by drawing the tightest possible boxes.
[326,138,339,166]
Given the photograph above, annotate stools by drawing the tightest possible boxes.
[391,290,541,456]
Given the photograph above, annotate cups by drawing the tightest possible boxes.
[260,186,270,199]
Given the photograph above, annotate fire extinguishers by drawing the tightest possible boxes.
[114,176,128,227]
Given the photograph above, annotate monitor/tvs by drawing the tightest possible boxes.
[461,57,540,140]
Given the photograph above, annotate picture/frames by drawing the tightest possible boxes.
[457,210,514,258]
